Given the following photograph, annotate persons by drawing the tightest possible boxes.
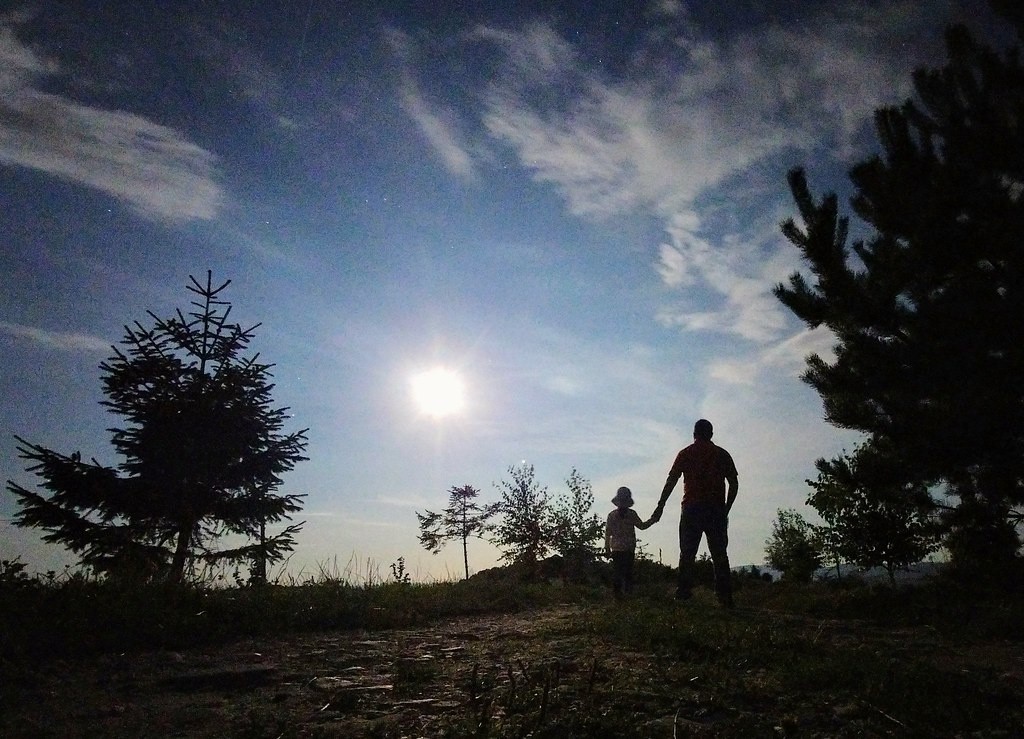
[652,420,739,609]
[605,487,657,599]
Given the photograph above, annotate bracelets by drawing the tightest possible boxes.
[658,501,665,506]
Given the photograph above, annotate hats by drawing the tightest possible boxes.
[612,487,634,508]
